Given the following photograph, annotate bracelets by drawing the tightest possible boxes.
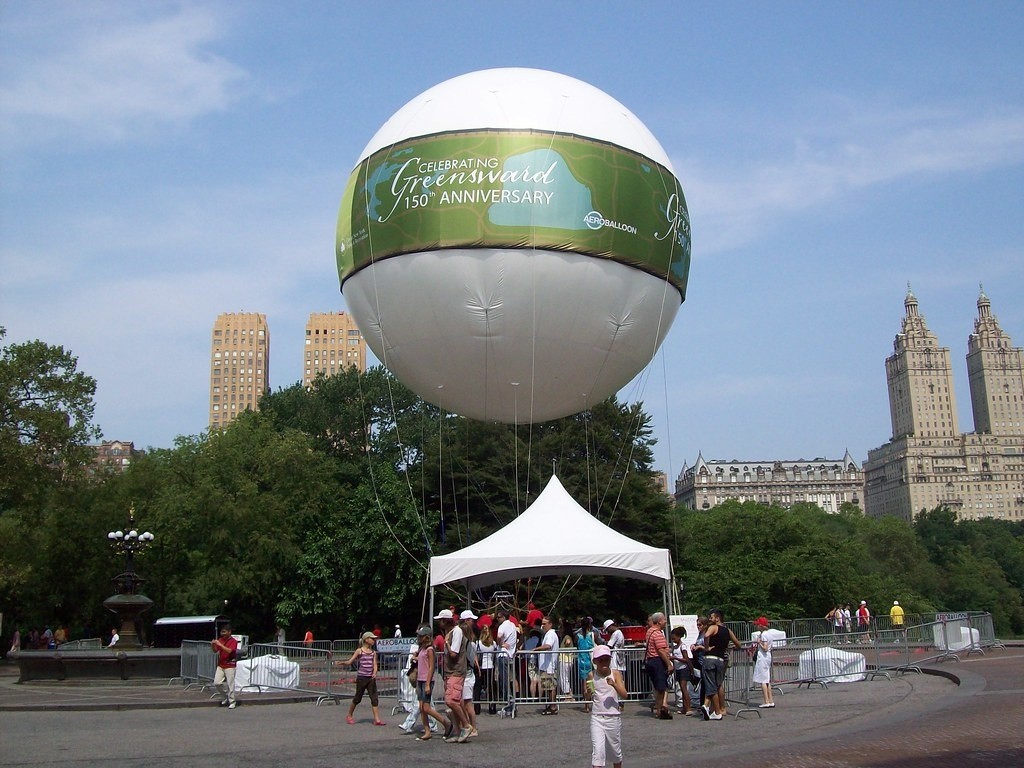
[758,642,760,644]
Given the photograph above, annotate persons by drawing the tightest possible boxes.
[335,632,387,725]
[391,625,401,657]
[668,627,694,715]
[855,600,875,644]
[10,624,70,652]
[586,645,628,768]
[890,601,905,643]
[398,603,627,743]
[275,624,285,655]
[360,624,382,639]
[687,610,741,720]
[211,624,237,709]
[107,628,120,649]
[303,628,314,659]
[636,612,683,720]
[753,617,775,708]
[825,603,852,645]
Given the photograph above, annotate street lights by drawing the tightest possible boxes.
[104,527,155,652]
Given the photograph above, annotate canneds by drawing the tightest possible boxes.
[586,680,594,693]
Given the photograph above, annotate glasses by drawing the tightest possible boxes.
[497,615,502,618]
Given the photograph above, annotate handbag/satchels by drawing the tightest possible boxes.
[477,640,483,666]
[641,659,648,673]
[407,659,418,688]
[753,649,758,661]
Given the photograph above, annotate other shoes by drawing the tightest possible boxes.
[398,720,479,743]
[345,715,354,724]
[693,675,702,691]
[759,702,775,708]
[497,710,512,716]
[660,704,723,721]
[222,699,236,709]
[373,720,386,726]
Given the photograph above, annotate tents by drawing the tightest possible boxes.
[420,474,682,647]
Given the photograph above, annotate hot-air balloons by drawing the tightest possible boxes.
[335,69,690,621]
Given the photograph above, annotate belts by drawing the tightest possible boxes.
[703,656,724,661]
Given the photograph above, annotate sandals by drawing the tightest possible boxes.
[541,706,558,715]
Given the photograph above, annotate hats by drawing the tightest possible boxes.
[433,609,453,618]
[603,619,614,631]
[753,617,768,626]
[860,601,866,605]
[460,610,478,619]
[845,602,853,606]
[593,645,613,658]
[362,632,378,640]
[416,627,432,637]
[894,601,898,604]
